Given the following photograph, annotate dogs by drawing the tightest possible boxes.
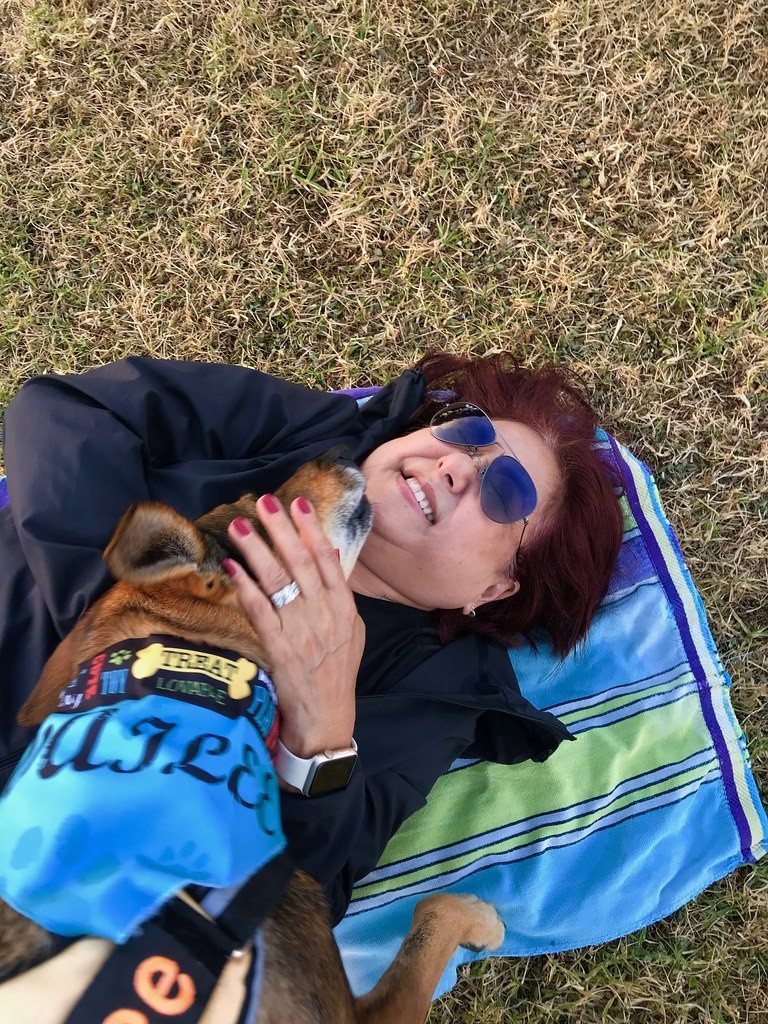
[0,458,509,1024]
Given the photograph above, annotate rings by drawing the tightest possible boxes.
[270,579,301,609]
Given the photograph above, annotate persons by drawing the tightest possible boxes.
[0,350,624,983]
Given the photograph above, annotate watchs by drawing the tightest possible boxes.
[275,737,358,800]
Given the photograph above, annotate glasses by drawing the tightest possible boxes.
[429,402,537,565]
